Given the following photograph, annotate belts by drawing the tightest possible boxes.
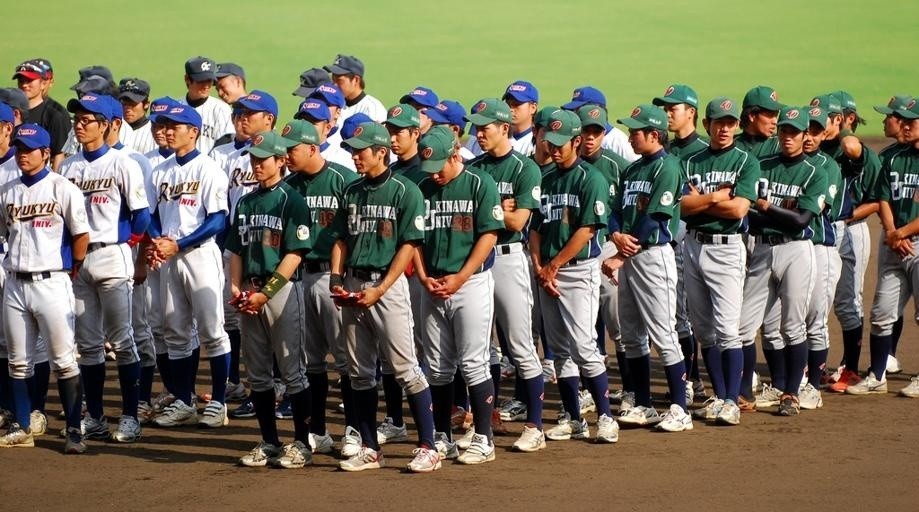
[353,270,387,282]
[305,262,331,273]
[754,236,784,246]
[86,242,106,250]
[249,277,266,288]
[8,270,50,283]
[642,244,648,250]
[502,245,510,254]
[569,258,577,265]
[687,230,727,244]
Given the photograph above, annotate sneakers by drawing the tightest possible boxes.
[540,359,558,385]
[433,432,459,462]
[341,426,363,457]
[450,388,657,473]
[274,441,312,467]
[0,395,142,456]
[498,355,516,380]
[404,446,442,471]
[657,352,919,433]
[139,379,294,429]
[338,447,385,471]
[240,441,285,466]
[309,430,335,453]
[377,417,407,443]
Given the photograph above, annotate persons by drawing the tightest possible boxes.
[2,55,918,472]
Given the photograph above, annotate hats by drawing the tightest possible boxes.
[873,97,919,119]
